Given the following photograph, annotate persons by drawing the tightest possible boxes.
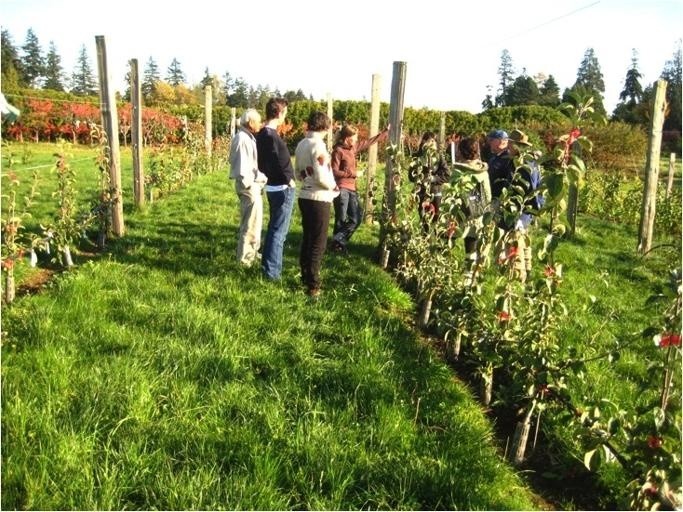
[501,130,543,289]
[254,96,296,287]
[291,110,340,299]
[444,138,491,293]
[408,132,449,235]
[225,108,265,270]
[330,125,390,250]
[479,129,516,266]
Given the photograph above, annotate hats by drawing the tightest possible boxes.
[488,129,532,146]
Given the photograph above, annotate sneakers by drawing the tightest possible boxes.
[308,287,319,296]
